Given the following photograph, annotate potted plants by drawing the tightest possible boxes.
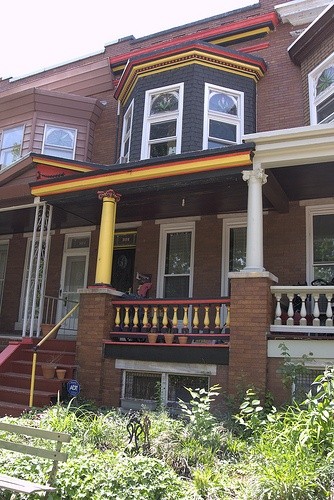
[178,336,188,344]
[164,327,175,344]
[147,327,158,343]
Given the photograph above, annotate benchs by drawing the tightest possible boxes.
[0,423,72,500]
